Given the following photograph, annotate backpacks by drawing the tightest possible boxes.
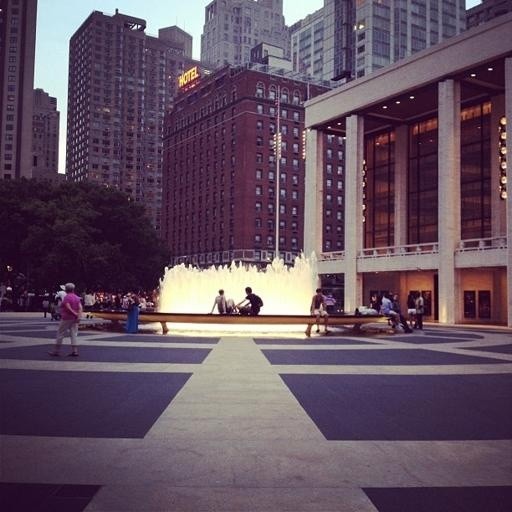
[253,295,263,307]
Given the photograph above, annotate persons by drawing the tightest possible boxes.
[310,288,425,332]
[47,282,82,356]
[12,284,158,320]
[232,286,263,315]
[209,289,237,315]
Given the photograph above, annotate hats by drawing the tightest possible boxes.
[59,285,65,291]
